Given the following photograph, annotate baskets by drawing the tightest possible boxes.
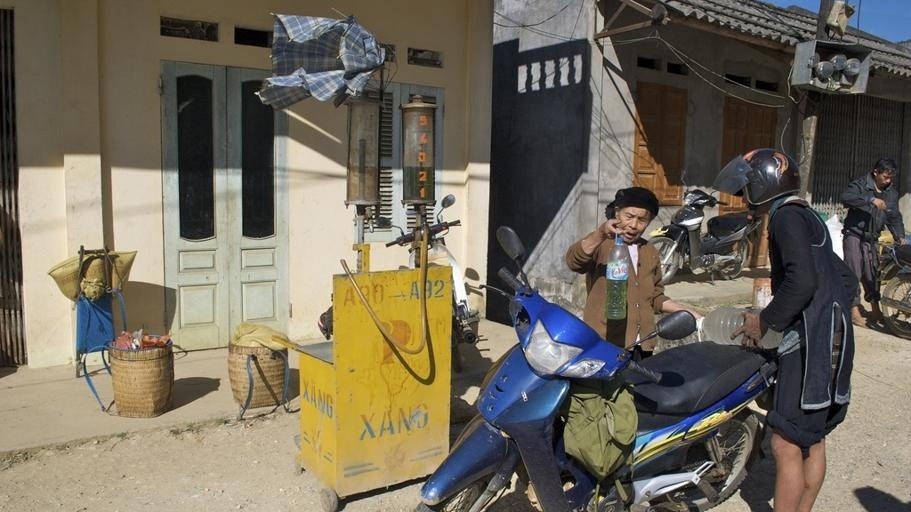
[228,341,289,410]
[109,335,174,418]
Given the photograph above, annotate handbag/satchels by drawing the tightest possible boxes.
[565,376,639,482]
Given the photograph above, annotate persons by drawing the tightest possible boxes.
[712,147,854,512]
[839,158,906,329]
[566,187,703,364]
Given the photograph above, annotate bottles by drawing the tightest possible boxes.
[606,228,630,320]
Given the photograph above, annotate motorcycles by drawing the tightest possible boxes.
[647,191,762,284]
[414,220,778,512]
[880,243,910,338]
[376,194,477,373]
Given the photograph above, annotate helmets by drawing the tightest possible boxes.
[712,148,801,210]
